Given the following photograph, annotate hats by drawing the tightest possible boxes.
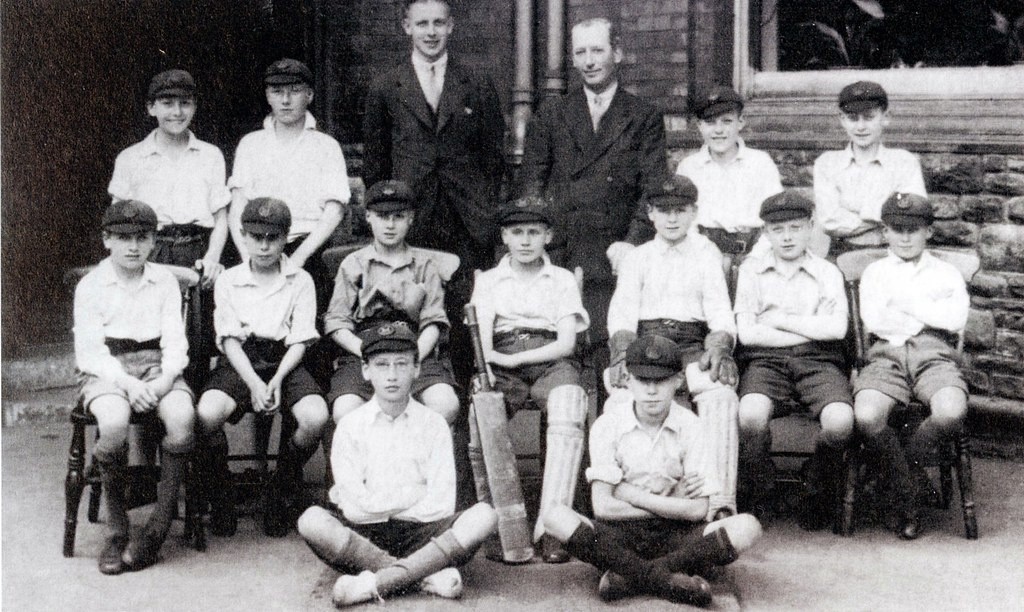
[881,192,934,227]
[361,323,418,362]
[265,58,313,86]
[627,336,682,380]
[148,69,197,99]
[839,82,889,113]
[500,196,551,225]
[102,200,158,234]
[365,180,415,212]
[646,173,699,208]
[693,84,745,121]
[241,196,293,236]
[759,190,812,224]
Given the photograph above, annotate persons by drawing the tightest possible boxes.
[225,58,351,393]
[606,174,739,520]
[732,191,856,530]
[513,17,671,355]
[813,80,929,289]
[674,86,783,306]
[461,196,592,564]
[323,178,461,427]
[196,198,331,537]
[541,331,764,608]
[296,323,498,608]
[73,198,197,575]
[851,193,971,539]
[109,66,233,468]
[362,0,511,414]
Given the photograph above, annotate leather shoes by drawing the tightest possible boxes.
[540,532,571,563]
[483,532,503,562]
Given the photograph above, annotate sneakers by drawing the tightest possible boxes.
[419,566,463,599]
[333,571,386,608]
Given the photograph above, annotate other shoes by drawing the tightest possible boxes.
[598,569,632,601]
[742,450,941,542]
[122,533,161,570]
[663,571,712,609]
[264,473,303,537]
[99,534,127,574]
[209,482,237,537]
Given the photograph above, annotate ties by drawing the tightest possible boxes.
[591,96,603,132]
[423,65,440,113]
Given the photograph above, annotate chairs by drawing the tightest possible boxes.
[325,233,461,488]
[593,239,734,417]
[472,263,586,475]
[735,246,862,511]
[55,250,208,558]
[839,244,986,542]
